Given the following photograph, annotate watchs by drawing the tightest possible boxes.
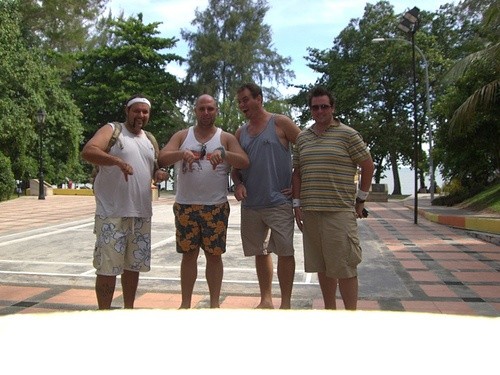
[355,197,365,204]
[157,167,168,177]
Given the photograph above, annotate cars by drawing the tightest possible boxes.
[228,183,235,192]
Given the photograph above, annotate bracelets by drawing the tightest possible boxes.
[357,190,369,200]
[293,199,301,208]
[213,145,226,159]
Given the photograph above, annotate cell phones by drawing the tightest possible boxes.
[362,208,369,217]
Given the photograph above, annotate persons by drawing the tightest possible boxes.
[157,93,251,309]
[83,95,169,309]
[292,87,373,311]
[230,83,303,309]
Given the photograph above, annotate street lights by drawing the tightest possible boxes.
[396,7,422,223]
[372,38,434,205]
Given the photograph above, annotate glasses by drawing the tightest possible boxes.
[311,104,332,111]
